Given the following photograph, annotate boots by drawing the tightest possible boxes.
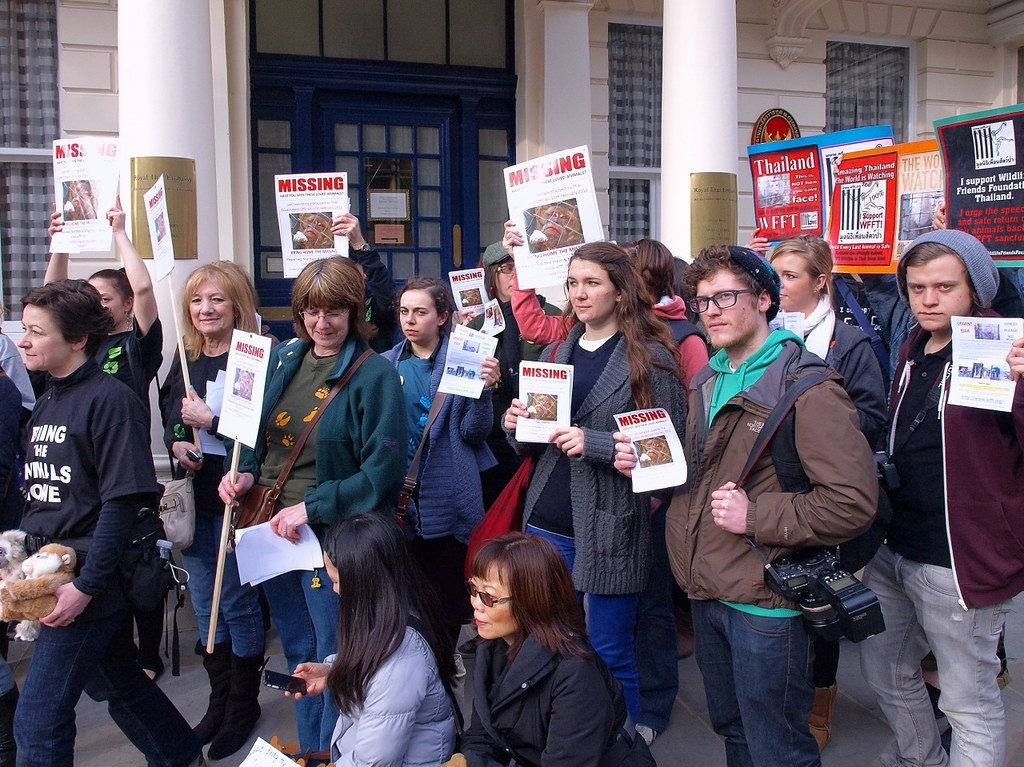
[804,680,839,757]
[192,638,233,746]
[207,651,271,760]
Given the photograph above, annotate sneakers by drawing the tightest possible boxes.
[635,723,659,747]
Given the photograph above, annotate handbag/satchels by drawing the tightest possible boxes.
[155,470,197,553]
[228,482,281,547]
[461,454,538,595]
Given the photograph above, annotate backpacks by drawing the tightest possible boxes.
[771,403,895,578]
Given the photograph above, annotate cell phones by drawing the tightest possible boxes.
[186,449,200,463]
[263,669,307,695]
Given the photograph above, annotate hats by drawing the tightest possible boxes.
[895,230,1000,309]
[725,246,781,322]
[483,241,510,269]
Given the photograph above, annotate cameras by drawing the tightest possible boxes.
[872,443,901,493]
[763,544,887,645]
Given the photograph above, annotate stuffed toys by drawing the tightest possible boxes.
[1,529,78,644]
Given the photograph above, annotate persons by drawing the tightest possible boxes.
[857,230,1024,767]
[438,532,657,767]
[503,220,712,751]
[227,211,1024,400]
[12,278,209,766]
[162,263,276,762]
[44,194,165,686]
[761,233,891,757]
[281,512,458,767]
[376,276,503,733]
[502,243,690,730]
[215,255,407,753]
[613,243,881,767]
[0,302,56,767]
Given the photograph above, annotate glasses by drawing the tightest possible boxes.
[687,288,759,315]
[465,578,515,607]
[497,265,515,274]
[298,309,350,322]
